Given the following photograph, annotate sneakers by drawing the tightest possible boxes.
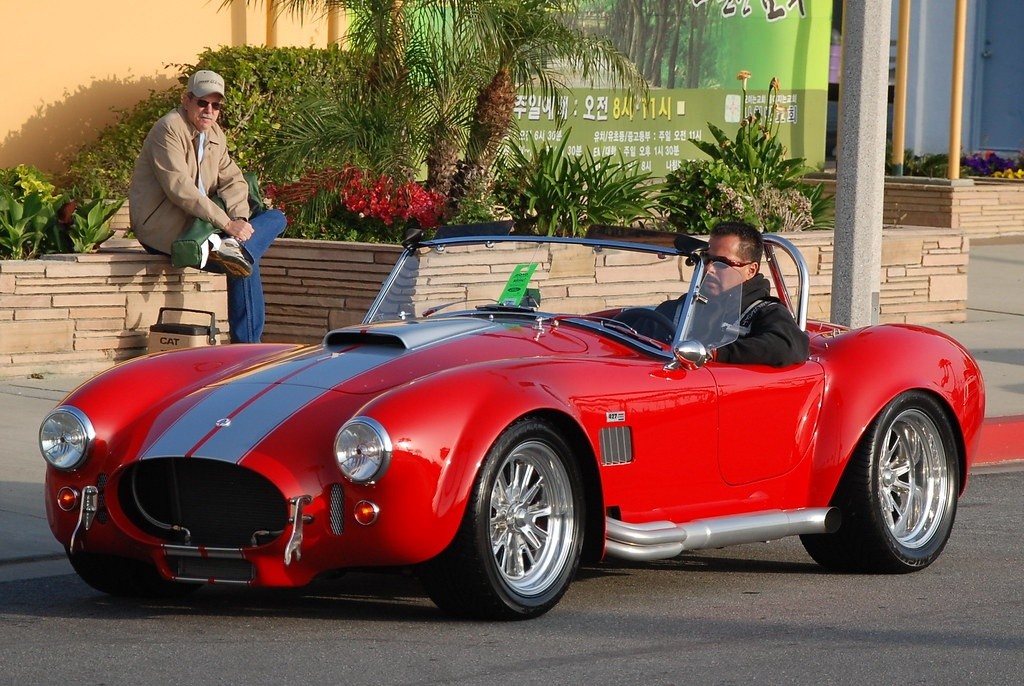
[206,238,251,277]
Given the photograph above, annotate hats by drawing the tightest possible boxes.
[187,69,225,97]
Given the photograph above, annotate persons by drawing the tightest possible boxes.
[129,70,287,345]
[632,220,809,368]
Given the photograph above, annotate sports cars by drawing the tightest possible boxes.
[37,230,989,621]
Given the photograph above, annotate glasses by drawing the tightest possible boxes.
[704,253,754,268]
[189,95,224,110]
